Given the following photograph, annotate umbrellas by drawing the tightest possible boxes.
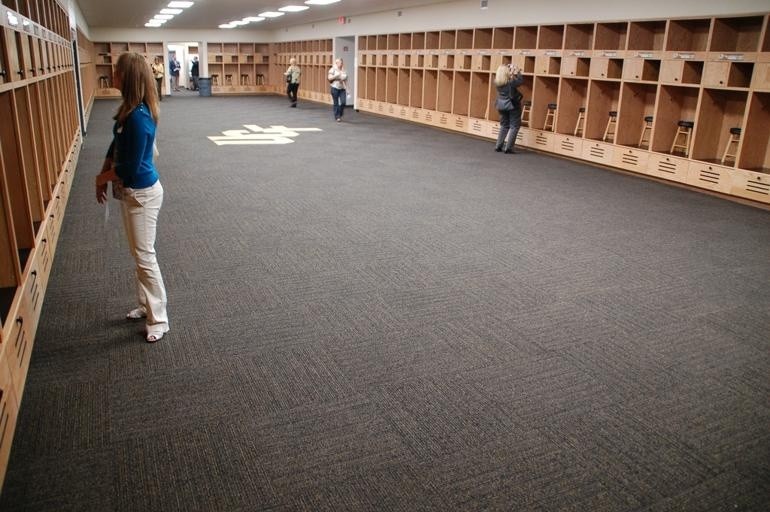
[504,147,513,154]
[495,146,503,153]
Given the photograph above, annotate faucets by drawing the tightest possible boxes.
[147,331,163,342]
[127,308,146,319]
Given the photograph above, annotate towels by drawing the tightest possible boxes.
[198,78,211,96]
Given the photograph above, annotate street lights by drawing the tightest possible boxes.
[496,96,515,111]
[327,79,335,84]
[286,74,292,82]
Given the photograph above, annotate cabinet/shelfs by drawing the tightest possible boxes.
[452,21,596,164]
[355,28,457,134]
[581,10,770,207]
[72,26,96,134]
[272,38,352,107]
[210,42,271,96]
[93,41,165,100]
[0,1,82,495]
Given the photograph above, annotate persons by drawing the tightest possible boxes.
[151,57,164,100]
[283,58,301,107]
[328,58,348,122]
[169,55,181,91]
[494,64,525,154]
[191,56,199,91]
[95,52,171,343]
